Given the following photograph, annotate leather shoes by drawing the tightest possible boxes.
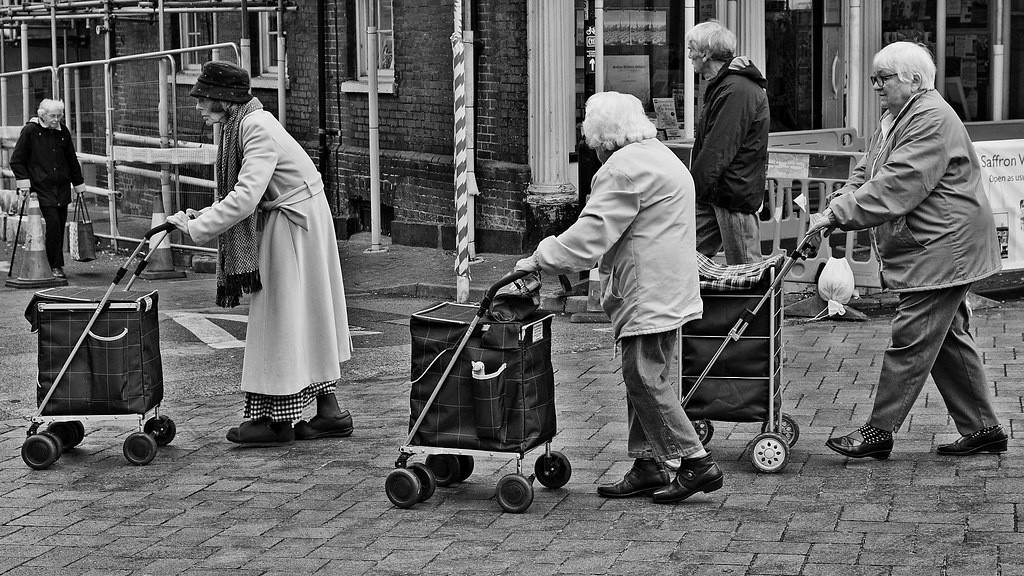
[225,416,295,448]
[292,410,354,440]
[937,424,1008,456]
[597,457,671,498]
[51,267,66,278]
[651,448,723,504]
[826,430,894,461]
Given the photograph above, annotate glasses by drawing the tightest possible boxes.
[870,70,907,87]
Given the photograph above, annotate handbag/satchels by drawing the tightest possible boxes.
[68,192,98,262]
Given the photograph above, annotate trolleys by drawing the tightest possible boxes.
[674,228,832,471]
[387,263,574,515]
[21,215,196,468]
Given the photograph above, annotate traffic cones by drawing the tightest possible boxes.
[139,196,188,281]
[4,191,68,288]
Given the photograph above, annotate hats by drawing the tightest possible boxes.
[189,61,253,104]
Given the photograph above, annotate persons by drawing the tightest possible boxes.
[686,20,772,269]
[804,41,1008,460]
[9,100,86,281]
[164,61,355,447]
[515,91,723,505]
